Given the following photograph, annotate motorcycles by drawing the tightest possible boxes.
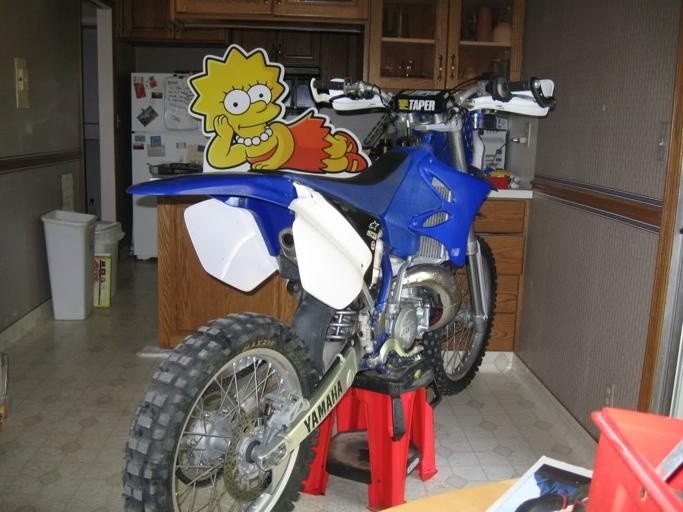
[122,72,557,512]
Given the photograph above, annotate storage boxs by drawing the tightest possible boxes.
[586,406,683,512]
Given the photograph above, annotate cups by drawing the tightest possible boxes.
[488,175,508,188]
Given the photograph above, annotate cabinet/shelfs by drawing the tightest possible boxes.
[117,0,233,49]
[167,0,370,35]
[353,0,528,99]
[157,194,532,356]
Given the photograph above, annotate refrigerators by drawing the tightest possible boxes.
[130,71,204,261]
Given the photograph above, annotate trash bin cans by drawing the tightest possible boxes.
[41,209,125,321]
